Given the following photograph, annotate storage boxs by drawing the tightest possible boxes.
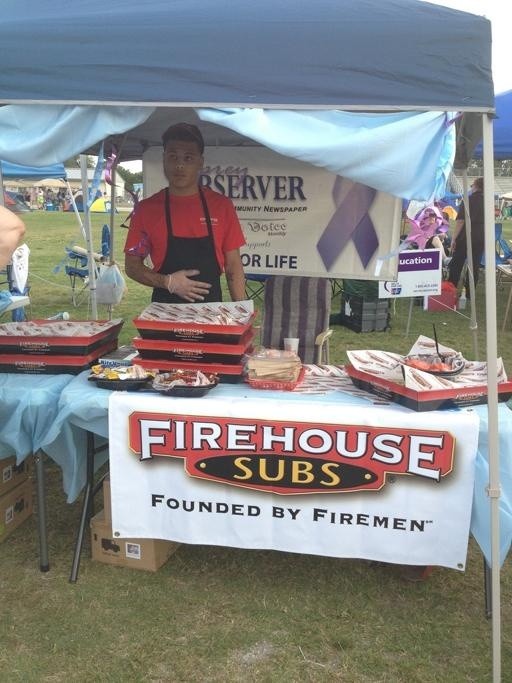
[1,449,36,545]
[88,478,185,573]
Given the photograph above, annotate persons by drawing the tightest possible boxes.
[124,119,252,304]
[6,178,144,211]
[446,175,485,301]
[409,207,453,269]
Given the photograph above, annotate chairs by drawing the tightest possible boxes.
[0,263,31,323]
[253,276,332,365]
[65,224,112,309]
[480,223,510,285]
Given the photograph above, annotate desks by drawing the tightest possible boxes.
[496,264,512,332]
[67,368,512,619]
[1,372,73,573]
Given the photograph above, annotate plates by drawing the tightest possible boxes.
[402,352,467,377]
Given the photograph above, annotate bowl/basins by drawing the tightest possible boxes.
[87,368,155,391]
[153,370,220,398]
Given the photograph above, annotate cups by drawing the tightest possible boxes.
[284,337,299,357]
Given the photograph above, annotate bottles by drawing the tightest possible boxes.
[459,288,466,310]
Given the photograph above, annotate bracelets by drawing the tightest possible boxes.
[166,272,173,290]
